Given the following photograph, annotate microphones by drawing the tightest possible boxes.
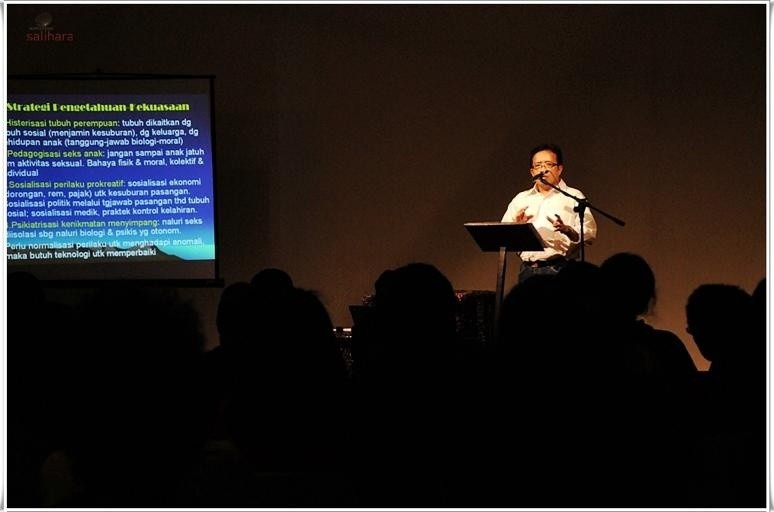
[532,171,549,181]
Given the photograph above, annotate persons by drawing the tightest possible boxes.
[6,248,766,508]
[499,143,598,287]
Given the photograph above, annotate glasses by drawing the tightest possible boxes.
[533,162,555,170]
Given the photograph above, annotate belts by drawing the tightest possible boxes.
[529,261,545,268]
